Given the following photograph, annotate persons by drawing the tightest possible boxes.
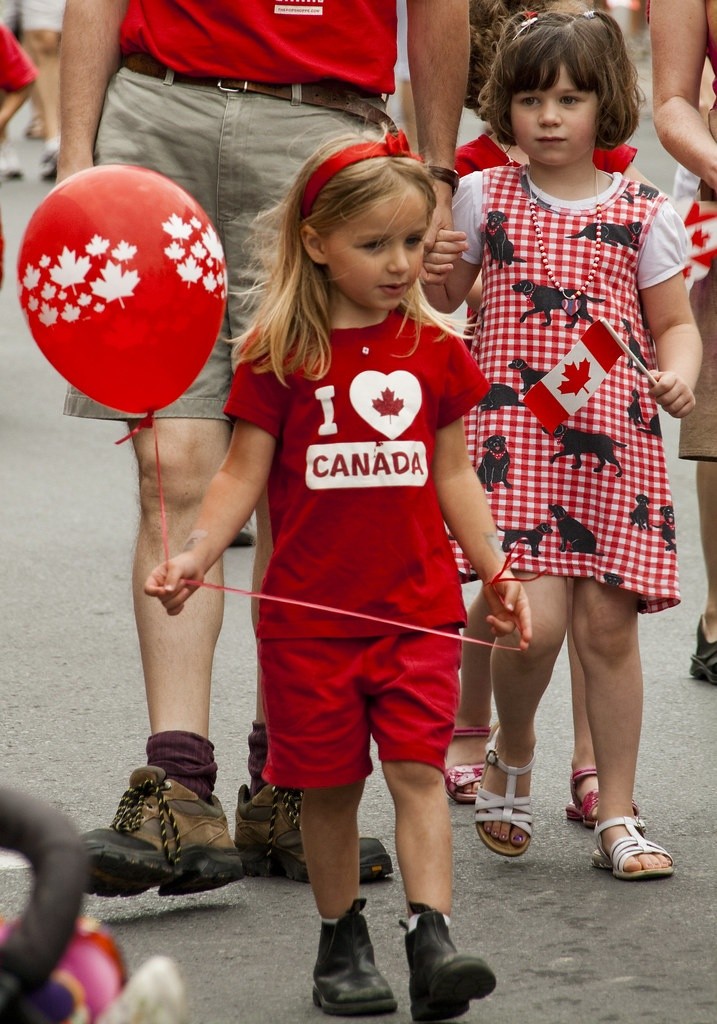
[0,0,66,295]
[419,5,704,881]
[445,0,666,829]
[646,0,716,687]
[142,130,533,1024]
[53,0,398,897]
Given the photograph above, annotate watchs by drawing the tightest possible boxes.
[428,165,459,197]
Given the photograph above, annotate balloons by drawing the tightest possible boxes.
[13,164,229,414]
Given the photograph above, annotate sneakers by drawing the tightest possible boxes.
[232,777,395,888]
[74,764,246,902]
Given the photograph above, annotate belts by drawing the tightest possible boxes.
[121,50,402,139]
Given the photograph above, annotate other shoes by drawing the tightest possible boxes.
[688,616,717,689]
[227,516,257,547]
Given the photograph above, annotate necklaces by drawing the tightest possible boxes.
[526,163,602,317]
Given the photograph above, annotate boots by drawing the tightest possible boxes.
[311,895,399,1017]
[399,904,497,1022]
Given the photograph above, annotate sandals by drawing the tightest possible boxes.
[590,815,676,880]
[443,725,496,805]
[565,769,641,829]
[473,720,537,857]
